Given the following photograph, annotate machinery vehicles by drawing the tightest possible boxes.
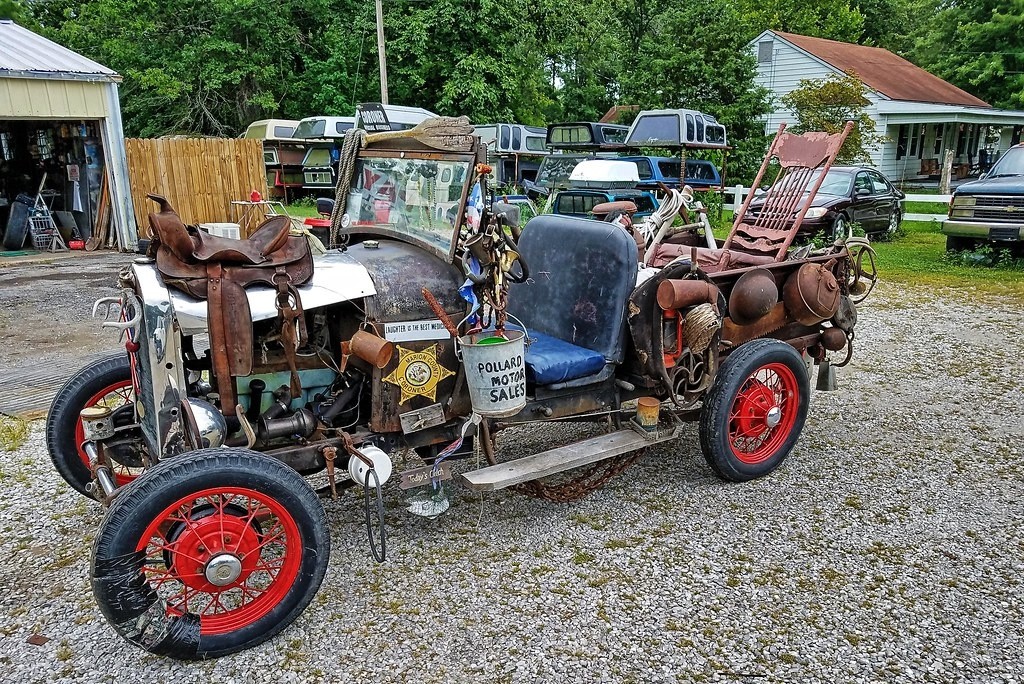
[45,121,875,660]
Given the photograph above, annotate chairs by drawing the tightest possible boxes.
[653,119,852,277]
[967,151,984,177]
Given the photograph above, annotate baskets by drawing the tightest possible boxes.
[681,302,720,354]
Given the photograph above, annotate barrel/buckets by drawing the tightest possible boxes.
[454,307,532,418]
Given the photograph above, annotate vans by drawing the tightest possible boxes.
[243,116,357,140]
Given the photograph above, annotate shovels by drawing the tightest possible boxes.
[84,167,108,252]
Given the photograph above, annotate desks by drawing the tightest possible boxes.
[41,194,62,209]
[229,199,298,231]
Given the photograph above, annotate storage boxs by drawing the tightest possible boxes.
[32,233,59,249]
[206,222,241,241]
[27,215,54,233]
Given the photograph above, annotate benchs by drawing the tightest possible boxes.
[919,157,944,179]
[485,212,639,390]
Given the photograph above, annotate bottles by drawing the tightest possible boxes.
[81,121,86,136]
[90,123,95,136]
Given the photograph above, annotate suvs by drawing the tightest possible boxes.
[941,141,1024,266]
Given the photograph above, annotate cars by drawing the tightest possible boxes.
[734,166,906,245]
